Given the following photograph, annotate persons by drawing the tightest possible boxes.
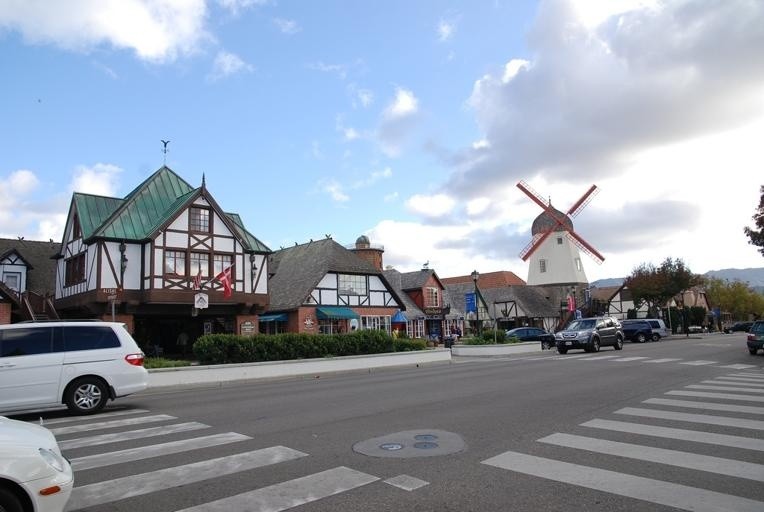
[451,325,461,338]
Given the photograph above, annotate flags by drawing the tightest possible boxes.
[193,272,202,290]
[215,266,232,300]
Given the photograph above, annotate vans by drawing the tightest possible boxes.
[621,318,668,343]
[0,321,149,416]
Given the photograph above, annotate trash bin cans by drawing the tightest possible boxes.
[444,338,455,349]
[538,334,552,350]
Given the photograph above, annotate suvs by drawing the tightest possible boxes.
[556,315,624,355]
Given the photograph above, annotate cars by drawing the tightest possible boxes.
[505,327,555,350]
[744,320,764,354]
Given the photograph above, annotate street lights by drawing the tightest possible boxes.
[471,269,480,338]
[680,290,689,337]
